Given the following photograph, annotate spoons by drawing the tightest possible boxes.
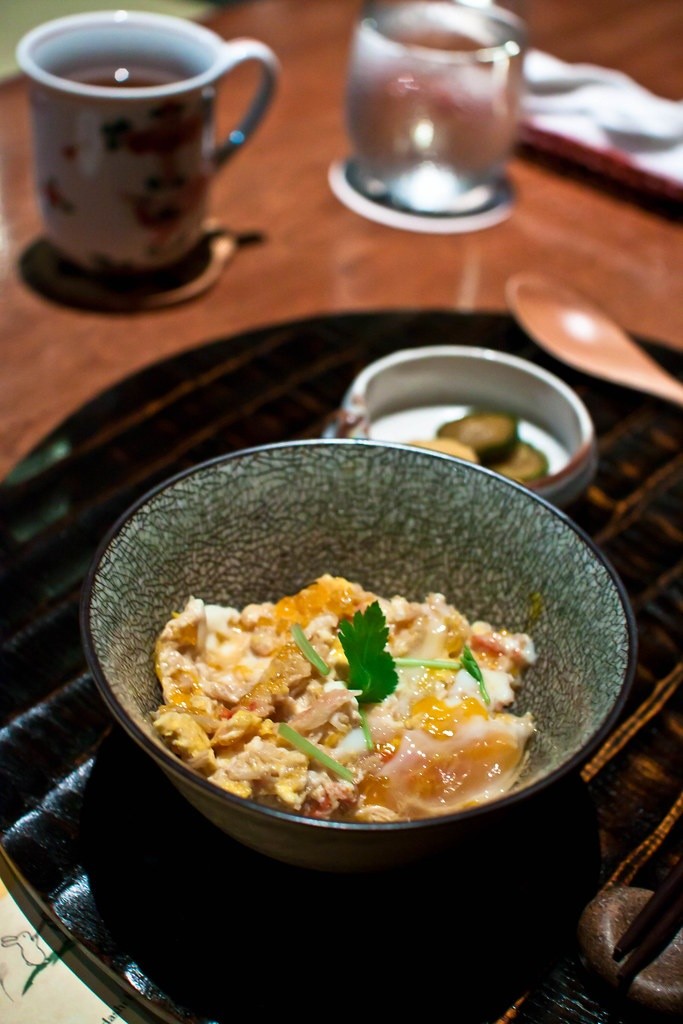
[504,271,683,406]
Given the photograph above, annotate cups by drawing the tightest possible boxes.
[16,9,280,272]
[343,1,529,215]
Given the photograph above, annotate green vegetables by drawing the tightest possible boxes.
[276,599,491,781]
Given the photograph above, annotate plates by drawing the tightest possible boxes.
[335,345,598,509]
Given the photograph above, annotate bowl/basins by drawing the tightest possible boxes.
[79,439,639,874]
